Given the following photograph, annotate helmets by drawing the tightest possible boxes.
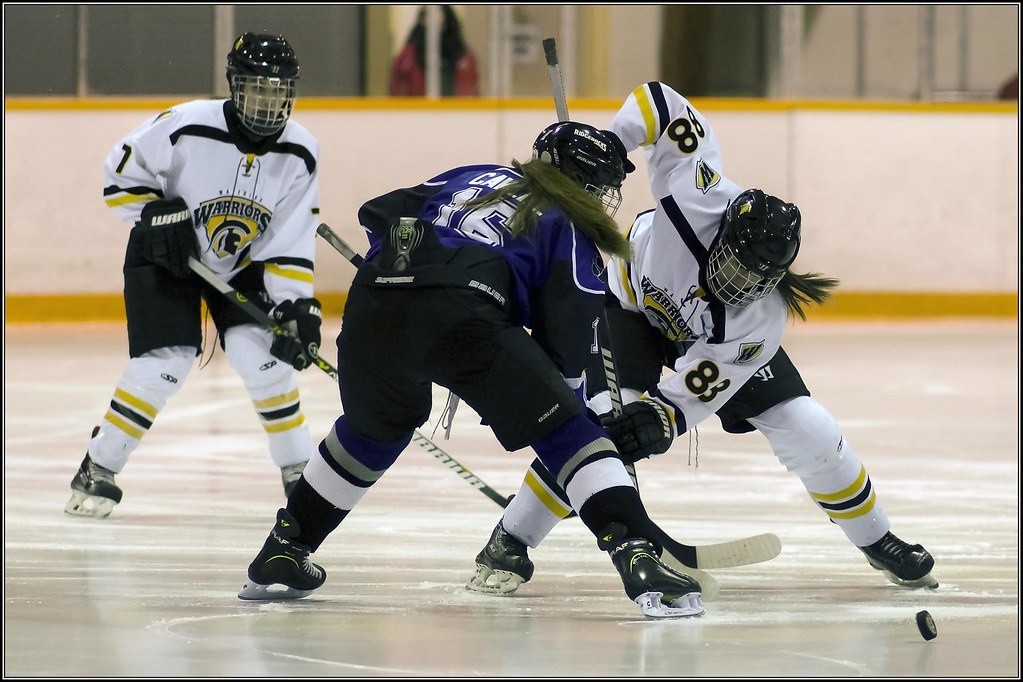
[226,30,299,137]
[532,121,625,219]
[706,189,801,309]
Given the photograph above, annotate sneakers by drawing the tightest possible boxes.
[596,522,706,618]
[281,462,306,498]
[237,508,326,601]
[65,426,122,518]
[856,530,938,589]
[466,494,535,594]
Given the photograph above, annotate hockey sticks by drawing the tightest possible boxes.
[542,33,785,571]
[189,255,510,508]
[314,220,724,607]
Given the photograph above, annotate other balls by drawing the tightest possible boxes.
[915,610,939,642]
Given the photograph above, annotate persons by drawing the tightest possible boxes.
[467,81,938,594]
[388,5,478,96]
[237,120,706,618]
[66,35,322,517]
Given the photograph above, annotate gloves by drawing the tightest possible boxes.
[270,297,323,372]
[141,196,201,279]
[598,396,674,465]
